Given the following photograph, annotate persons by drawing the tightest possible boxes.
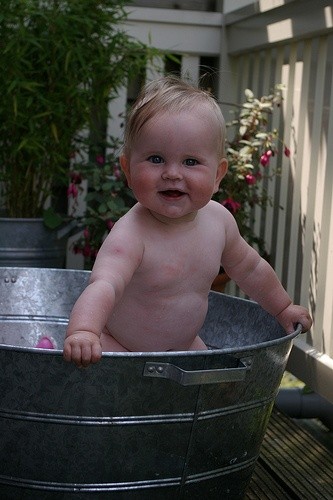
[63,75,314,365]
